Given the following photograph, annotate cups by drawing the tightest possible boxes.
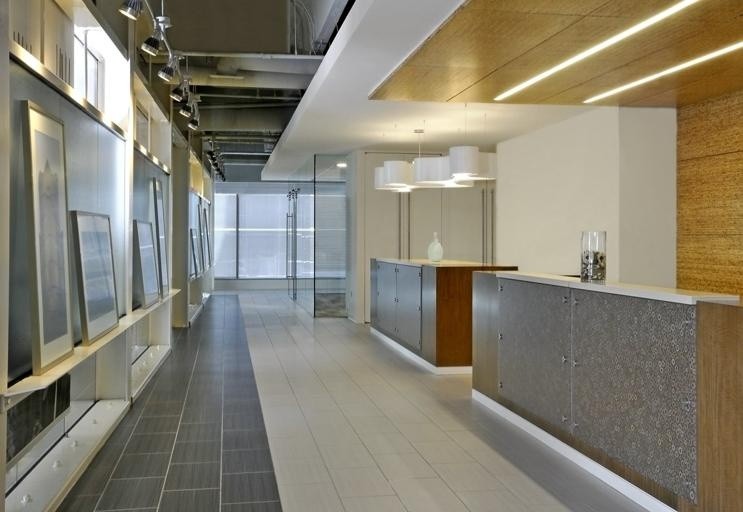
[580,229,607,287]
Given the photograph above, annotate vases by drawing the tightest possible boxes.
[427,231,443,262]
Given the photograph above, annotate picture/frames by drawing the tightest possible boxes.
[189,202,212,279]
[70,210,120,346]
[133,219,160,310]
[19,98,74,377]
[153,176,170,301]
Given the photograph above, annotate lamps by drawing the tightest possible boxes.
[203,139,226,183]
[116,0,201,131]
[373,102,497,193]
